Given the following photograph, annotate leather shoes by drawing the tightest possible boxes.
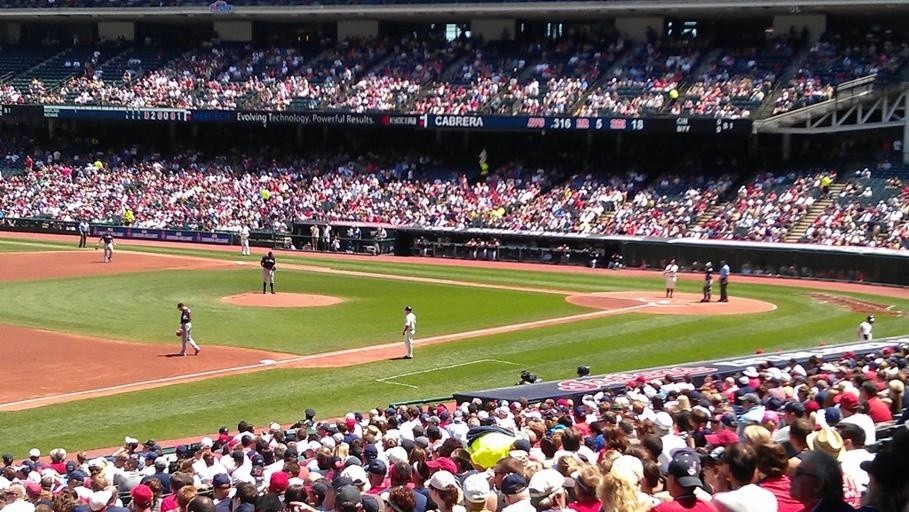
[718,298,723,302]
[724,299,728,302]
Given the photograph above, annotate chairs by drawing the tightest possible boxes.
[2,39,909,209]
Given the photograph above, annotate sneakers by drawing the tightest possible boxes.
[195,348,200,356]
[705,299,710,302]
[178,353,187,356]
[701,299,704,302]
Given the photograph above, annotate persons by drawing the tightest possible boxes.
[259,251,276,294]
[698,261,714,303]
[717,259,730,302]
[1,23,909,272]
[663,258,679,299]
[175,302,201,356]
[0,344,908,512]
[402,306,417,358]
[855,314,876,342]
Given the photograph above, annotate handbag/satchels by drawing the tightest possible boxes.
[466,425,515,447]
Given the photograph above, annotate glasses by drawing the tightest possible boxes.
[795,468,815,478]
[494,472,510,477]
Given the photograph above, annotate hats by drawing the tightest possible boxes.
[738,346,908,427]
[131,485,153,506]
[611,455,644,484]
[412,407,490,503]
[805,428,843,459]
[501,474,527,494]
[860,452,908,479]
[230,408,415,512]
[126,436,230,468]
[664,451,703,487]
[522,411,542,420]
[529,469,575,497]
[625,372,739,445]
[212,473,232,489]
[1,448,112,512]
[745,426,770,445]
[868,315,875,322]
